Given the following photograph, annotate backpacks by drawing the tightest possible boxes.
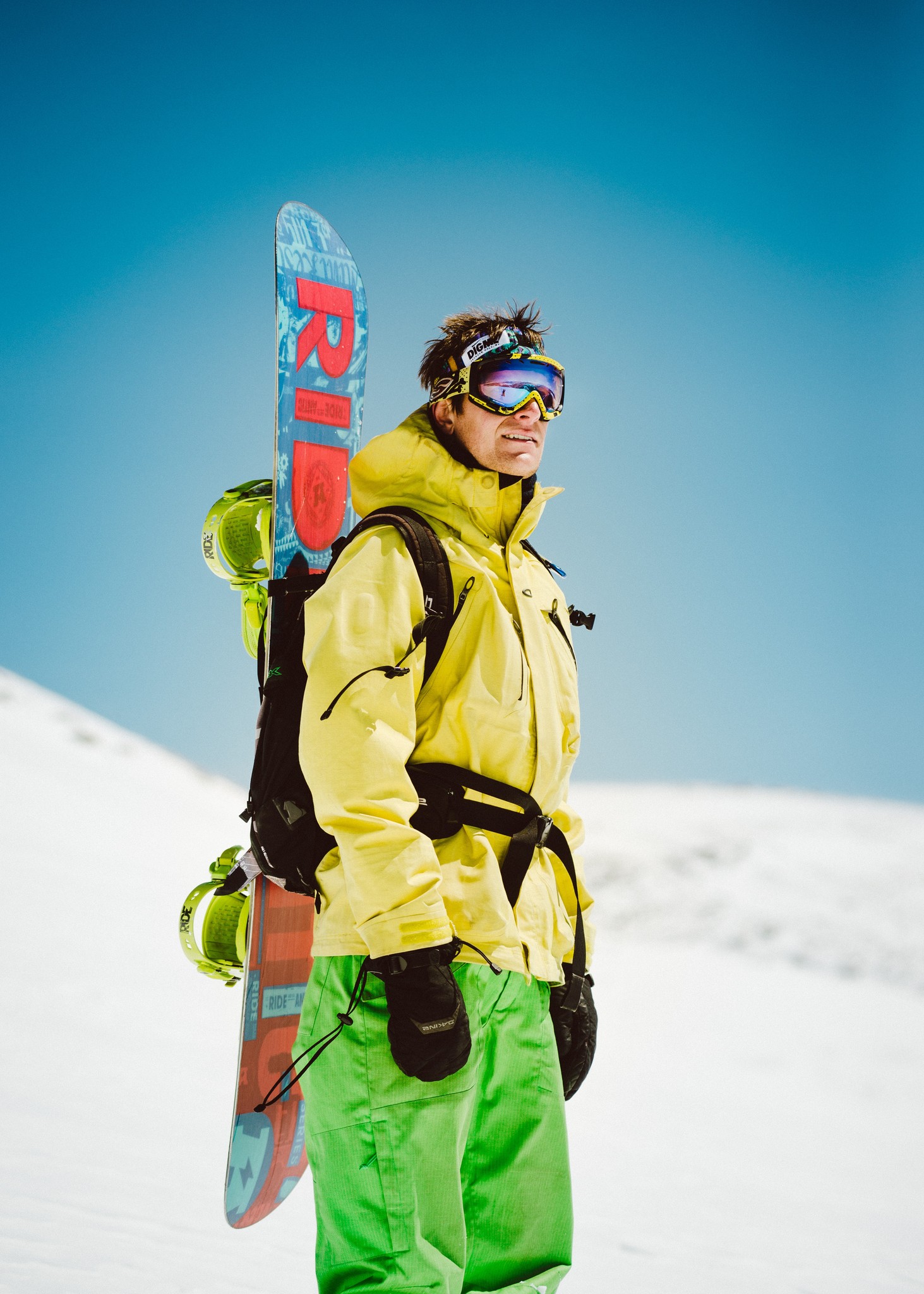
[236,504,461,914]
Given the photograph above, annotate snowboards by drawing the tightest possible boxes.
[215,193,378,1233]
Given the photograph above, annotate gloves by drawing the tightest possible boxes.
[363,942,472,1083]
[548,960,599,1100]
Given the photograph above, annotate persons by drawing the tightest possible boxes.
[299,295,598,1294]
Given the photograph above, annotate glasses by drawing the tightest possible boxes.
[433,353,567,423]
